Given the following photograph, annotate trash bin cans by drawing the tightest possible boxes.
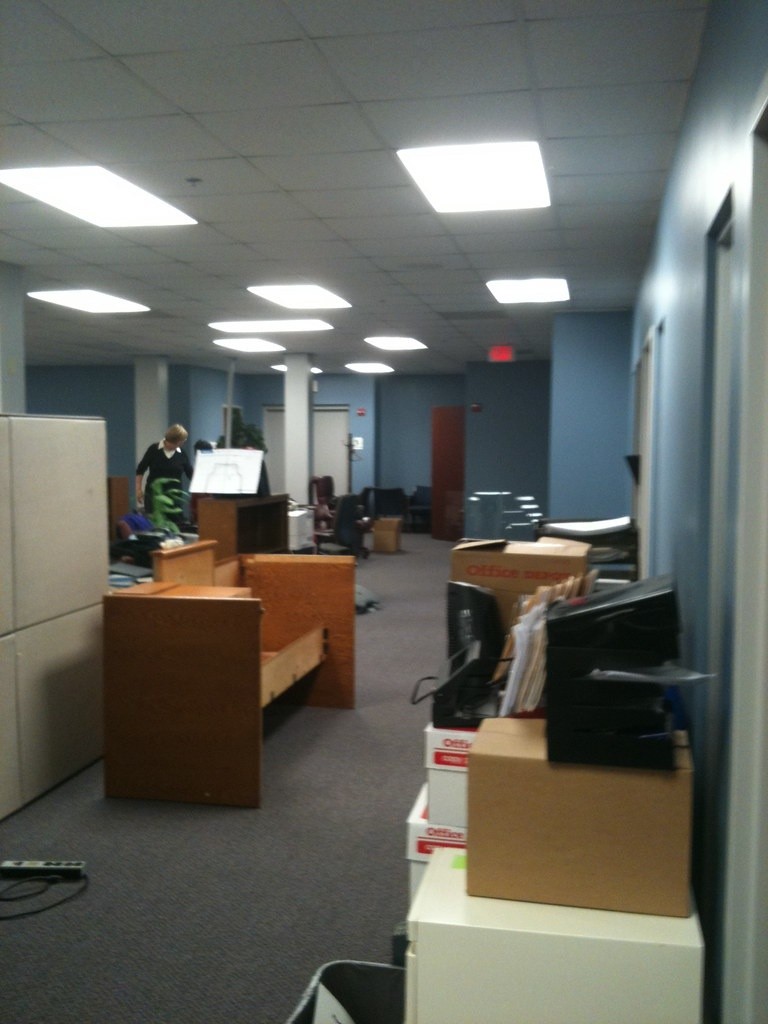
[286,959,407,1024]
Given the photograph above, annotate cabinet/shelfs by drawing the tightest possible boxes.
[198,492,292,569]
[0,414,109,820]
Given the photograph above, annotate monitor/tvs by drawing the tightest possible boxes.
[446,580,504,687]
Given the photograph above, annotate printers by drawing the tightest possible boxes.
[537,573,681,773]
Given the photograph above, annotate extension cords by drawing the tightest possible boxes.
[0,861,86,881]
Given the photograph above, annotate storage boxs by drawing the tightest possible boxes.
[373,516,401,552]
[546,747,681,771]
[428,768,466,826]
[406,780,467,860]
[544,713,677,748]
[466,718,691,918]
[549,681,662,710]
[424,696,499,773]
[451,535,592,633]
[541,575,687,674]
[406,848,703,1024]
[542,709,665,733]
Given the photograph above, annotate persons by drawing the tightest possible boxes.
[135,425,193,522]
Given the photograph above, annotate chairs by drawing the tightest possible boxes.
[317,475,337,508]
[313,491,362,547]
[376,487,409,530]
[409,485,433,530]
